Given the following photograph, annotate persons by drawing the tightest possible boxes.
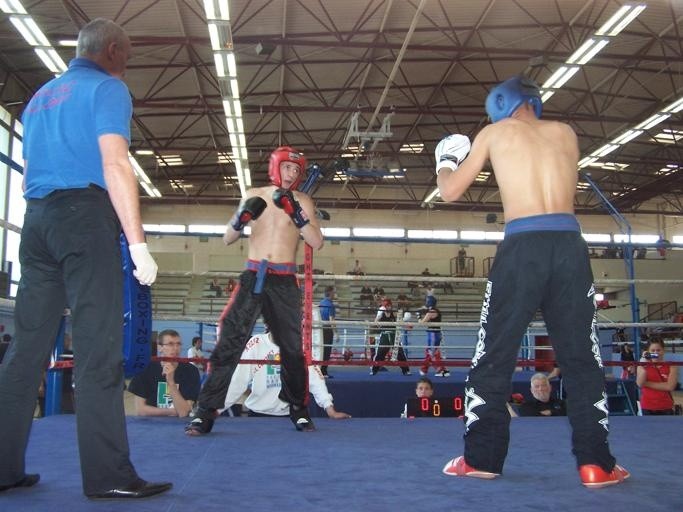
[351,259,363,275]
[129,330,200,419]
[401,378,441,419]
[0,16,175,499]
[620,343,635,379]
[214,323,350,420]
[421,284,434,298]
[315,284,338,380]
[637,338,677,416]
[416,295,450,377]
[396,288,412,310]
[421,267,432,281]
[419,267,429,276]
[373,284,386,304]
[225,274,236,296]
[456,247,466,272]
[188,335,206,373]
[330,347,341,359]
[518,372,567,417]
[206,273,220,298]
[369,299,412,375]
[0,331,13,363]
[549,367,565,391]
[359,282,373,305]
[434,76,631,490]
[184,145,324,435]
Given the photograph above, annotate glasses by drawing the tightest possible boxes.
[159,342,183,347]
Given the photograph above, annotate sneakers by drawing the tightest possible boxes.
[289,405,314,431]
[371,367,377,376]
[579,463,630,489]
[442,457,500,480]
[404,372,412,377]
[435,370,451,377]
[186,409,215,437]
[420,370,426,376]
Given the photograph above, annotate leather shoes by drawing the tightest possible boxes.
[323,372,336,380]
[1,474,41,492]
[89,478,173,497]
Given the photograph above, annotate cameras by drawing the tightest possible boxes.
[645,353,658,359]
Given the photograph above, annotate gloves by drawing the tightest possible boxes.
[335,333,341,342]
[232,196,268,232]
[421,307,429,314]
[434,134,472,174]
[130,243,160,287]
[416,309,424,319]
[272,189,310,229]
[402,312,412,322]
[377,307,387,319]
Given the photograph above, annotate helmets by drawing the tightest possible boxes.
[383,300,393,307]
[485,77,543,121]
[424,297,437,309]
[343,351,354,363]
[269,147,305,190]
